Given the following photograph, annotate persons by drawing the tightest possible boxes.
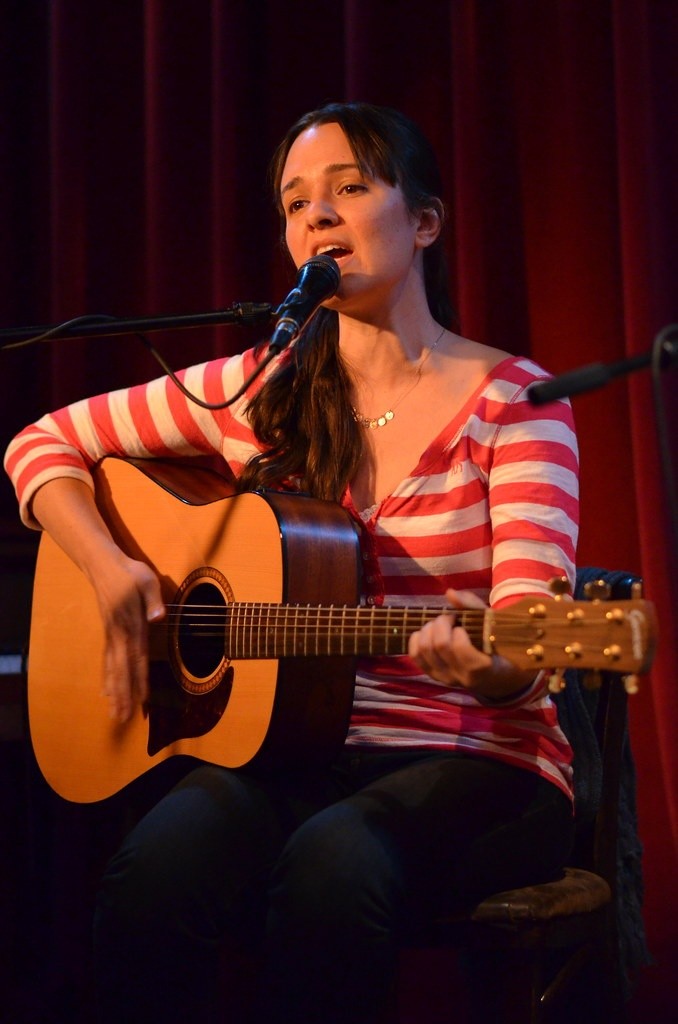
[1,103,588,1023]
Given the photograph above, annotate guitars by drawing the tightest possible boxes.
[26,455,660,805]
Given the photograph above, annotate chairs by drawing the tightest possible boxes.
[400,568,647,1023]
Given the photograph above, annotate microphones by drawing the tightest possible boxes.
[269,254,342,349]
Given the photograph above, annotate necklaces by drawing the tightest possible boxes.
[342,328,448,430]
